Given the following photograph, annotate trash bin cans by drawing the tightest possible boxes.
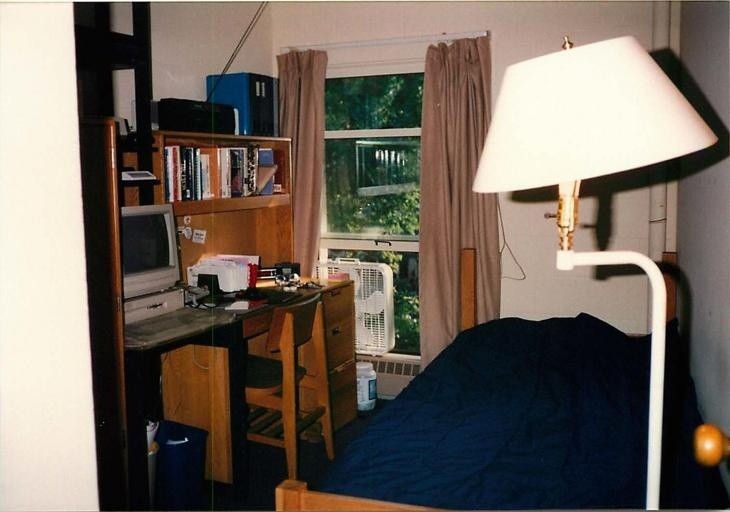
[152,419,208,511]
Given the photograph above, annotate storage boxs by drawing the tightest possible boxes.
[124,421,208,511]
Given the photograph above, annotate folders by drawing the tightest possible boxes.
[249,73,263,135]
[208,72,251,135]
[262,75,274,137]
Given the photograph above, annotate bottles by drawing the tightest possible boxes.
[356,362,377,411]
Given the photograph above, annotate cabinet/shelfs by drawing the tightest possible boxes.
[118,131,294,287]
[298,283,357,436]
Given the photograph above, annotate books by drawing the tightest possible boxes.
[164,145,276,203]
[187,253,259,293]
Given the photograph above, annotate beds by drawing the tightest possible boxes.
[275,248,729,511]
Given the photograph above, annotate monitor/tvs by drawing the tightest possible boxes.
[121,204,181,299]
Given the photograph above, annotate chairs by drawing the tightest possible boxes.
[244,301,335,482]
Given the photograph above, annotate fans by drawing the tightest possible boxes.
[313,258,396,356]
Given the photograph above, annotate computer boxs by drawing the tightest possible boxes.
[123,287,185,325]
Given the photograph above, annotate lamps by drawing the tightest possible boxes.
[472,34,717,506]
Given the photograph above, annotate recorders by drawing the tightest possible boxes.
[158,97,236,135]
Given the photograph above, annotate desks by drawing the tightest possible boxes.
[124,277,358,484]
